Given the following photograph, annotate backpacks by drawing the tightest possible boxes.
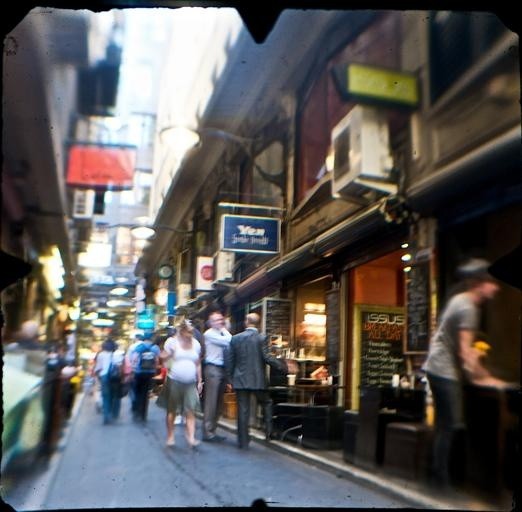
[141,343,157,369]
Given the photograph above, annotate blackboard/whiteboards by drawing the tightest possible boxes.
[402,255,436,354]
[351,304,408,413]
[324,290,342,363]
[261,297,295,355]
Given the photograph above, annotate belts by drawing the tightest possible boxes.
[206,361,224,367]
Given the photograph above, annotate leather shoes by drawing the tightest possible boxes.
[266,432,272,440]
[239,443,249,449]
[203,435,225,441]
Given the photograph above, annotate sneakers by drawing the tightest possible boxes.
[188,438,201,446]
[166,439,175,447]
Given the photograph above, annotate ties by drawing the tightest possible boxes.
[221,332,228,361]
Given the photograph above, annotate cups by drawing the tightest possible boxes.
[286,375,296,386]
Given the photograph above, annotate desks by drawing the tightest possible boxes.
[360,387,428,469]
[291,384,348,404]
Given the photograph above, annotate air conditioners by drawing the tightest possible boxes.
[329,103,390,202]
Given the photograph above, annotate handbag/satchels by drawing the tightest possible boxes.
[108,362,122,383]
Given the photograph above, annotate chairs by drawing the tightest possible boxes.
[275,403,347,450]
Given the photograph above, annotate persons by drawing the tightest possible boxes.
[7,319,69,374]
[203,310,233,445]
[421,270,509,492]
[157,318,203,448]
[226,312,300,449]
[91,309,204,428]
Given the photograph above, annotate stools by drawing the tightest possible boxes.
[382,420,433,480]
